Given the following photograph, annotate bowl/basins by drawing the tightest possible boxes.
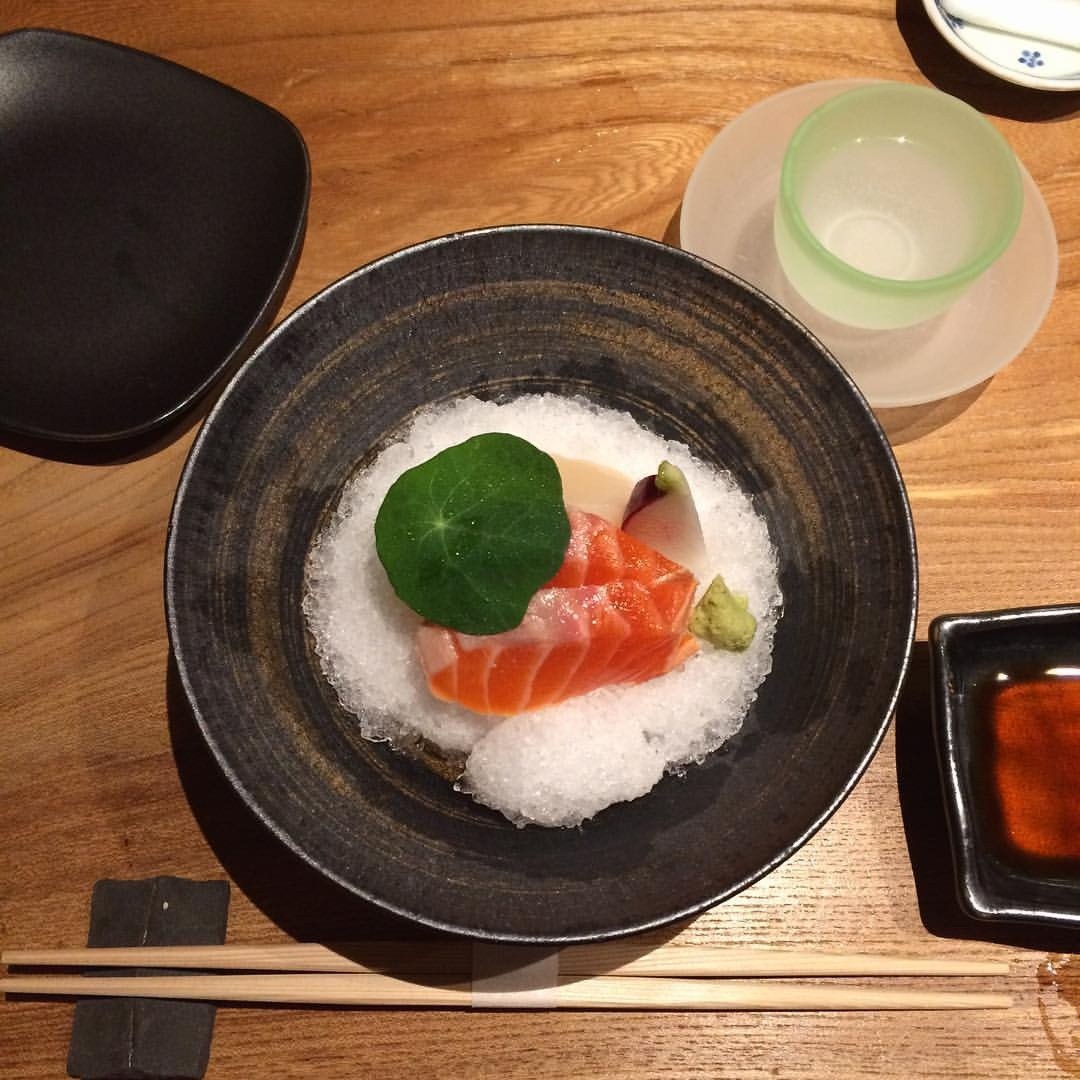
[167,228,921,942]
[928,603,1080,927]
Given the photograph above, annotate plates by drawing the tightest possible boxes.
[0,26,314,444]
[680,76,1060,408]
[922,0,1080,92]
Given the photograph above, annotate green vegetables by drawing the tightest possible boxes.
[375,433,570,639]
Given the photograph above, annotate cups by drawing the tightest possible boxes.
[771,80,1023,331]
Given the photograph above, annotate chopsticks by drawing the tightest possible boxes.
[0,939,1016,1012]
